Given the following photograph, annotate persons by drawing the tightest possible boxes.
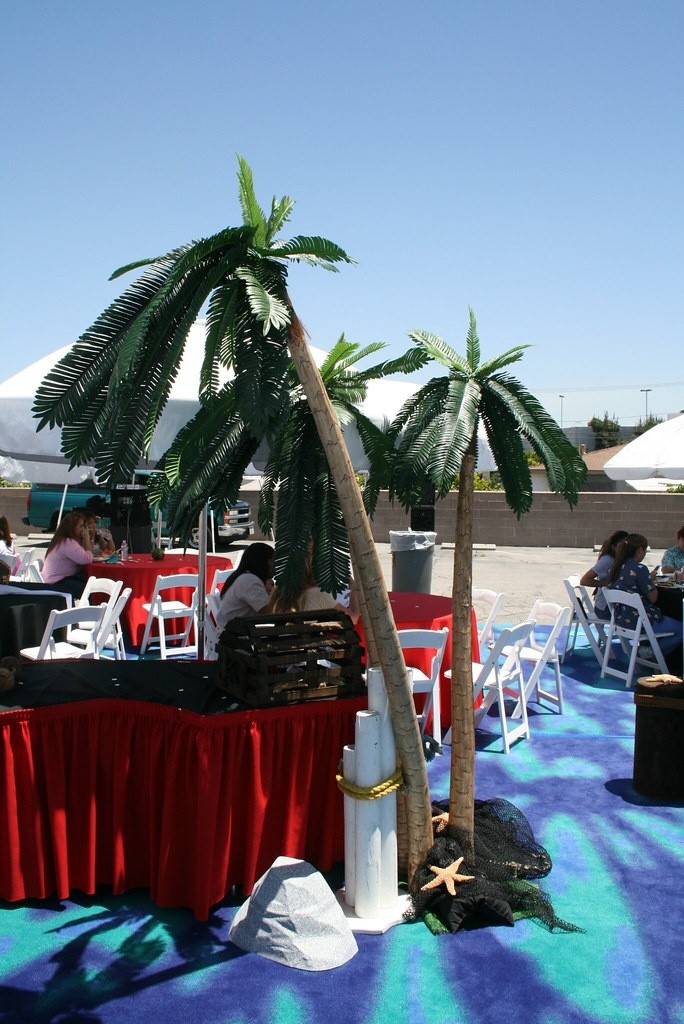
[215,539,360,632]
[580,531,630,621]
[662,526,684,574]
[0,511,115,601]
[605,534,684,662]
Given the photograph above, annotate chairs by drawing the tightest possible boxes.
[362,573,673,755]
[0,547,240,660]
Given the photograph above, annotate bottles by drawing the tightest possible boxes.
[94,528,113,540]
[120,540,128,561]
[674,566,684,585]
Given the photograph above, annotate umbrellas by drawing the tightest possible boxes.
[0,319,417,660]
[604,412,684,481]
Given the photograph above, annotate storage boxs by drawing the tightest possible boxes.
[216,610,364,706]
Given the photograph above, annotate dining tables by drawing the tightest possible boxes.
[652,576,684,623]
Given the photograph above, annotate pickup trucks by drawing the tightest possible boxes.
[25,463,249,551]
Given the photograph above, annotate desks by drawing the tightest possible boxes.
[0,659,370,918]
[80,553,231,647]
[0,583,75,659]
[353,593,482,738]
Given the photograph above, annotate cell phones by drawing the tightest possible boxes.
[654,566,660,571]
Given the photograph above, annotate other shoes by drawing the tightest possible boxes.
[639,646,654,673]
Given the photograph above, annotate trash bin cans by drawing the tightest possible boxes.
[389,530,437,594]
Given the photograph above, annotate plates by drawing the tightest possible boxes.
[93,556,109,561]
